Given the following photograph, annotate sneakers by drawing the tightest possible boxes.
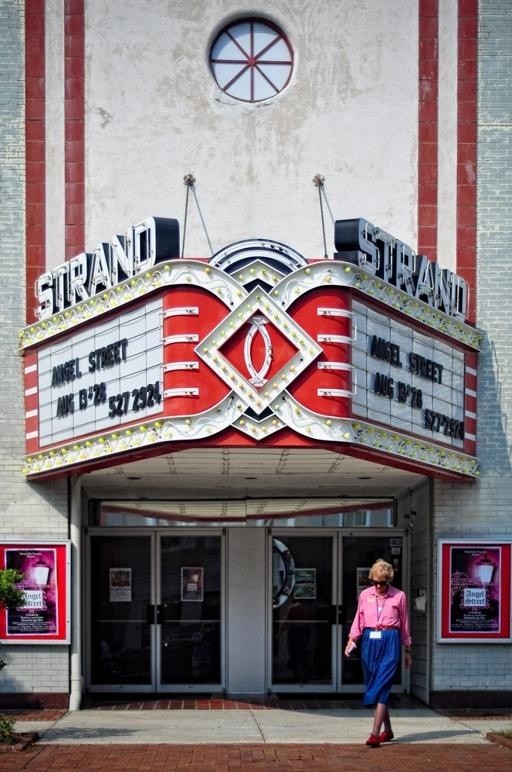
[365,730,394,746]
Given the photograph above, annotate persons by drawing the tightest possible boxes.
[344,558,414,748]
[275,600,313,684]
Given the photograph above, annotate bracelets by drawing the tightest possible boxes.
[404,649,411,653]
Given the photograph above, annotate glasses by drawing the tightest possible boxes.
[369,578,390,586]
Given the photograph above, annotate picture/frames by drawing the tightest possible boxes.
[435,537,511,645]
[1,538,73,645]
